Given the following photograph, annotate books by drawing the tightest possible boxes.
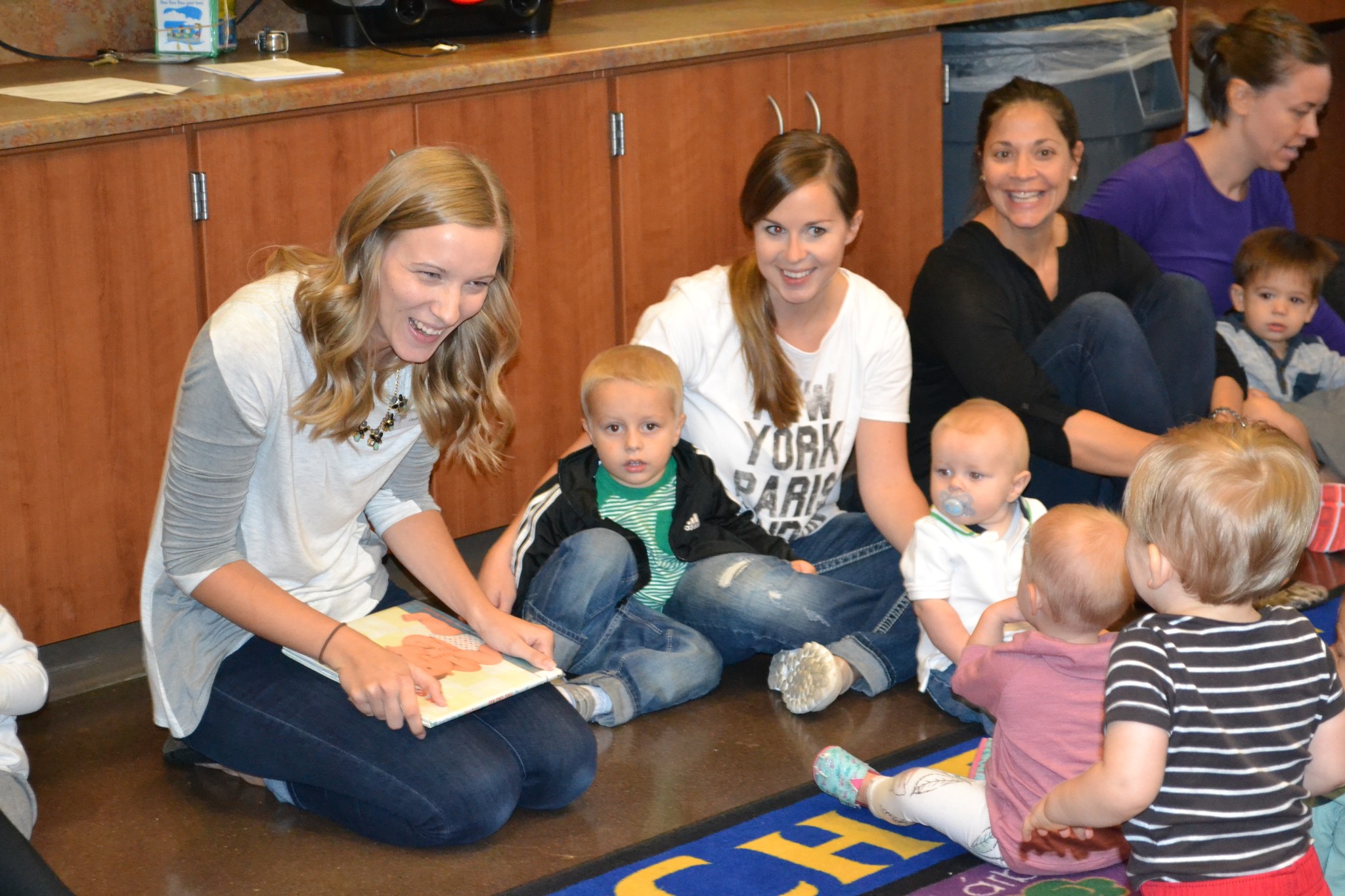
[282,599,568,728]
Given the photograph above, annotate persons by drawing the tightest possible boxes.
[900,398,1051,735]
[512,343,814,727]
[138,138,598,848]
[476,129,930,717]
[904,73,1251,515]
[811,503,1136,875]
[1215,227,1345,553]
[1079,6,1345,357]
[1023,422,1345,894]
[1,606,50,842]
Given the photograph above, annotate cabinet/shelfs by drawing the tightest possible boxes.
[196,78,617,542]
[610,33,944,344]
[1,131,203,650]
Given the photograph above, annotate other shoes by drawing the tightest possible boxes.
[554,683,594,723]
[967,737,992,779]
[768,641,853,714]
[813,745,882,809]
[164,733,217,767]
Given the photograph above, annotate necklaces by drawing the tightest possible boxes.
[353,368,409,450]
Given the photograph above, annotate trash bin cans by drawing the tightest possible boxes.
[941,1,1185,244]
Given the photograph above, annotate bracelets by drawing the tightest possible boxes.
[318,622,348,665]
